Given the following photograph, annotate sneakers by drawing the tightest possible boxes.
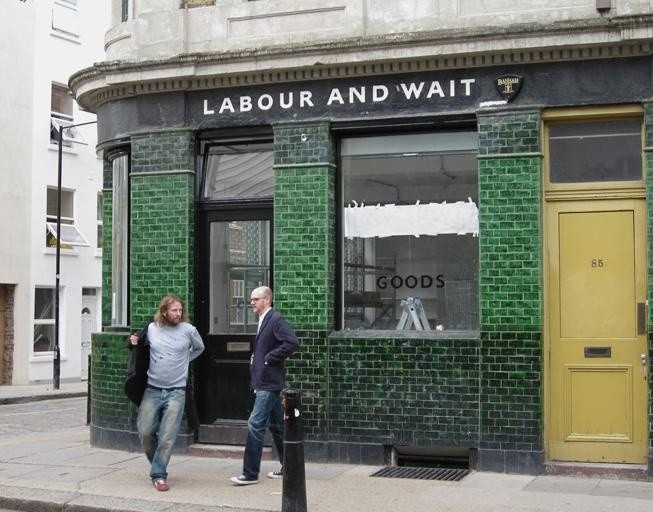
[230,474,259,484]
[154,480,169,491]
[268,471,283,478]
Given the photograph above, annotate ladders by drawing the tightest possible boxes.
[395,296,432,330]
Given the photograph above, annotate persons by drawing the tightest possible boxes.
[124,295,205,490]
[230,286,301,485]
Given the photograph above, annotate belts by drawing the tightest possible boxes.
[149,385,186,391]
